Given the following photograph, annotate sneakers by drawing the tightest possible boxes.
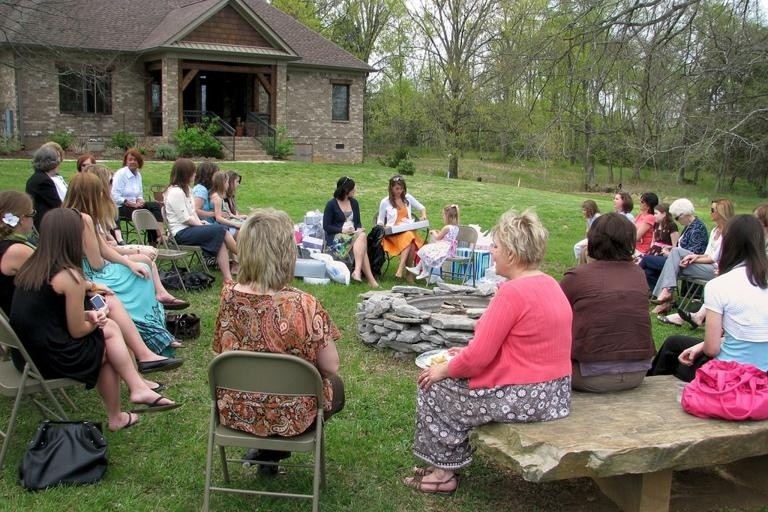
[139,357,183,374]
[151,381,167,392]
[405,266,420,276]
[416,272,430,280]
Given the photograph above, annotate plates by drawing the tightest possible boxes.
[415,349,450,369]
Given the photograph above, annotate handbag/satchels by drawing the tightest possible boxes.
[200,256,234,271]
[681,360,767,421]
[366,224,386,277]
[159,268,212,291]
[166,313,200,339]
[19,421,109,491]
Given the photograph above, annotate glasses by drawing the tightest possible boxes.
[392,176,404,182]
[674,213,683,221]
[24,209,37,218]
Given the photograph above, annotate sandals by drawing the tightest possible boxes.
[403,466,458,495]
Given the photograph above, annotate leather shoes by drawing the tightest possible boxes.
[242,448,291,478]
[650,292,699,329]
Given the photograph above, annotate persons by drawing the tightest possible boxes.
[640,204,680,257]
[553,210,657,394]
[571,198,601,259]
[635,198,705,299]
[162,157,249,286]
[401,202,574,495]
[657,203,768,330]
[8,207,181,431]
[208,207,343,478]
[376,174,429,286]
[578,191,637,265]
[647,198,733,316]
[639,214,768,384]
[0,140,192,394]
[404,202,460,280]
[322,176,386,291]
[629,192,658,262]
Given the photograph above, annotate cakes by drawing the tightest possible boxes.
[429,354,449,365]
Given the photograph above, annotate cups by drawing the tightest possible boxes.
[451,239,458,258]
[673,381,689,403]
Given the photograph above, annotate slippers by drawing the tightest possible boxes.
[121,396,183,430]
[162,298,189,310]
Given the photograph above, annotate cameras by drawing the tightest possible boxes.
[91,294,105,310]
